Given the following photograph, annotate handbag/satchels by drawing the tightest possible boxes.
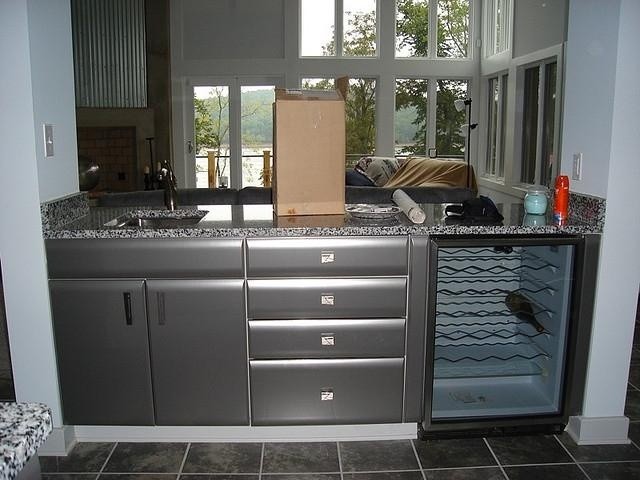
[446,195,504,223]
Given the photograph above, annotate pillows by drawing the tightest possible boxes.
[345,168,376,186]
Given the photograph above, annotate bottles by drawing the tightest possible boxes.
[553,175,570,221]
[506,293,545,333]
[523,184,550,216]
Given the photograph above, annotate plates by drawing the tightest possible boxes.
[346,206,403,219]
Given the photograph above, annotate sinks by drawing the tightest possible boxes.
[103,208,209,228]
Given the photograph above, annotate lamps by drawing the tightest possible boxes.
[454,97,478,201]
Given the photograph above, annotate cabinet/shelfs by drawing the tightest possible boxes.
[43,238,250,444]
[244,239,431,442]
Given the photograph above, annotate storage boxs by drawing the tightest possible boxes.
[272,73,349,217]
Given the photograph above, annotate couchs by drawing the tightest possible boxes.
[344,156,478,204]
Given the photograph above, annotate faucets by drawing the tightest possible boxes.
[156,159,180,212]
